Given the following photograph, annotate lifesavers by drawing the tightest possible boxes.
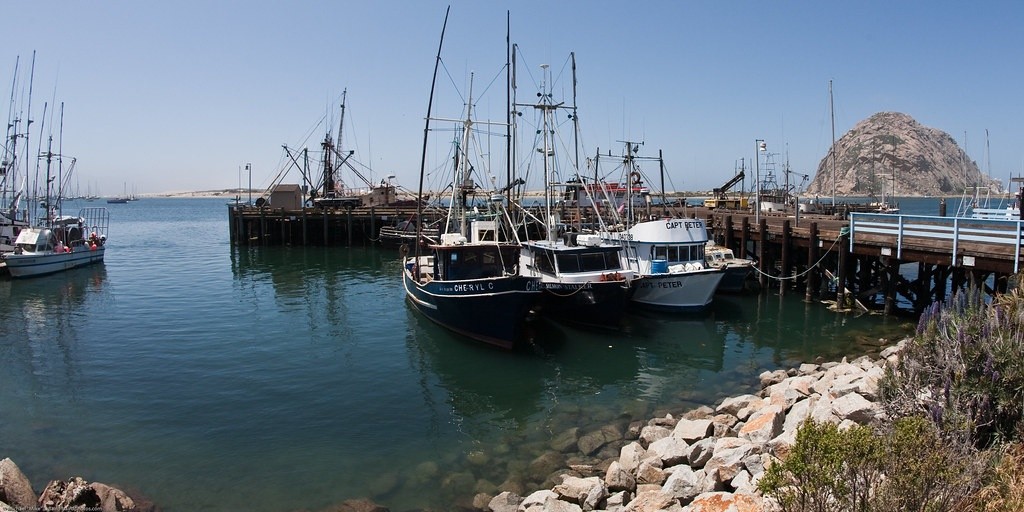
[399,244,410,254]
[631,170,640,184]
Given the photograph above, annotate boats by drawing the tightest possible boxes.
[590,138,729,310]
[703,226,755,296]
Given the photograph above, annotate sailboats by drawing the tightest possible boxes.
[961,128,1021,220]
[505,39,645,329]
[734,139,899,218]
[229,5,547,353]
[0,48,139,279]
